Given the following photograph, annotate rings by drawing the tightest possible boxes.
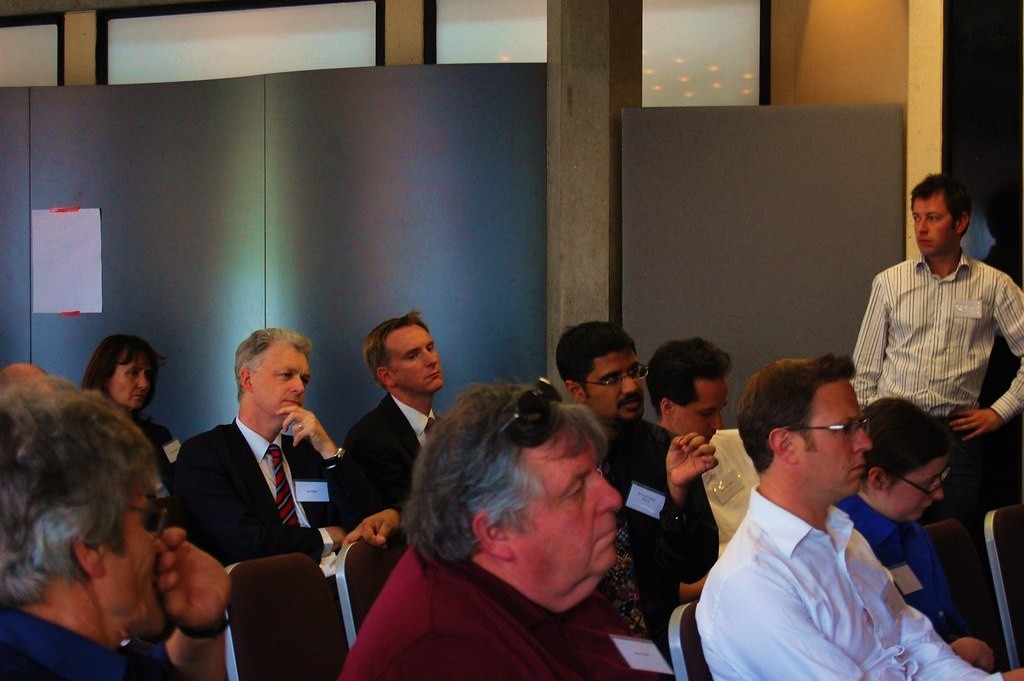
[298,423,305,430]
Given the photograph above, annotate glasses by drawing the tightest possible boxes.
[570,363,648,389]
[887,463,951,496]
[498,378,562,439]
[124,505,168,537]
[766,417,870,444]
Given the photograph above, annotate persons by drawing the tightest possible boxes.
[175,327,379,578]
[337,372,676,681]
[340,307,444,517]
[0,362,231,681]
[695,351,1024,681]
[82,335,179,533]
[644,334,761,559]
[851,173,1024,556]
[342,316,719,672]
[834,396,995,674]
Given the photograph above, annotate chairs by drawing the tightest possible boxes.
[214,484,1024,681]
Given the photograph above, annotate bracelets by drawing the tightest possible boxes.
[174,621,228,639]
[324,447,345,468]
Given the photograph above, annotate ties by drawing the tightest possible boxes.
[424,418,436,442]
[267,444,300,529]
[598,460,647,641]
